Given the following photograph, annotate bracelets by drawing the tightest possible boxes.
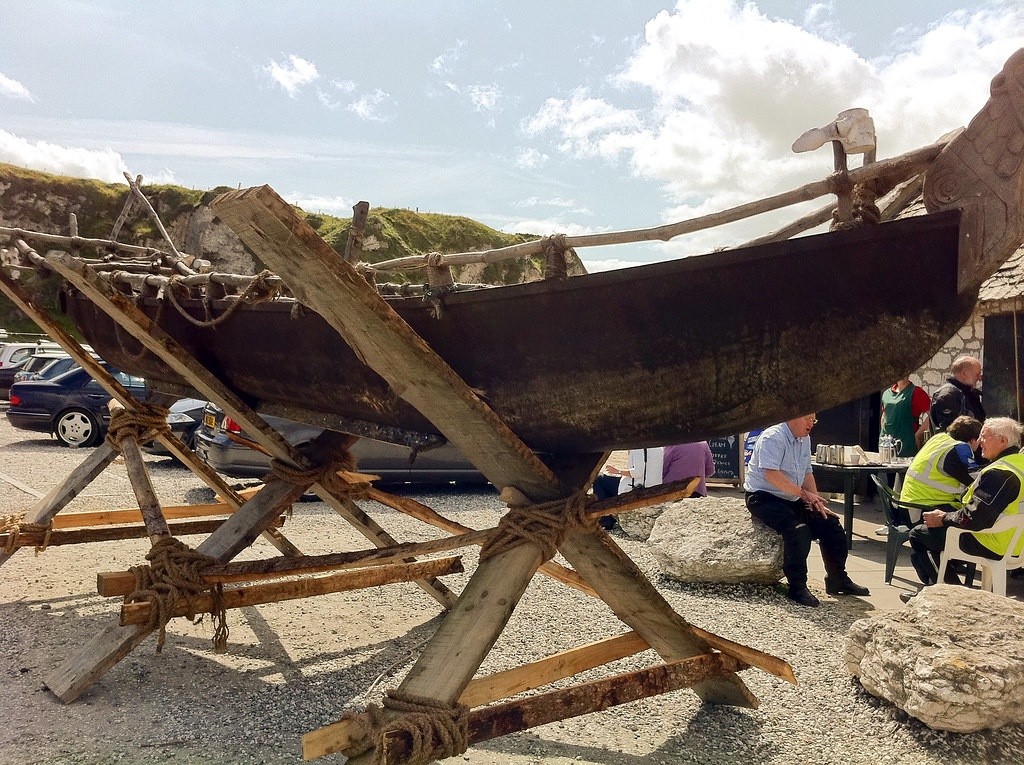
[619,469,621,474]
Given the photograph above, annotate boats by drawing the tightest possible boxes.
[52,47,1024,457]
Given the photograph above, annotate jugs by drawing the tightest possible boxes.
[878,434,902,464]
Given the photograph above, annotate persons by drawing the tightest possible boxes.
[898,415,982,574]
[899,418,1024,605]
[875,375,931,535]
[742,414,869,606]
[663,440,715,498]
[931,356,989,466]
[592,447,663,530]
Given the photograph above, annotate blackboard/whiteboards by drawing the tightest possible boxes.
[704,433,745,484]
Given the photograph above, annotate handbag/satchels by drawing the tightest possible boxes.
[628,482,645,491]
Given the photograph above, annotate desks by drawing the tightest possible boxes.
[811,463,910,550]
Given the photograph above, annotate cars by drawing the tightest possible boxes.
[195,398,493,505]
[0,328,186,450]
[133,390,208,456]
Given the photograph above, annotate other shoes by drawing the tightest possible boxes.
[788,582,820,606]
[602,518,617,530]
[826,577,869,596]
[875,525,889,535]
[899,584,923,605]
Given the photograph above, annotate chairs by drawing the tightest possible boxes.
[937,513,1024,598]
[870,473,957,586]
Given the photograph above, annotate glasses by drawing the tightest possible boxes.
[802,417,818,424]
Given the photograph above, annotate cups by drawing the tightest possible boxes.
[816,444,830,463]
[827,444,844,465]
[843,446,857,463]
